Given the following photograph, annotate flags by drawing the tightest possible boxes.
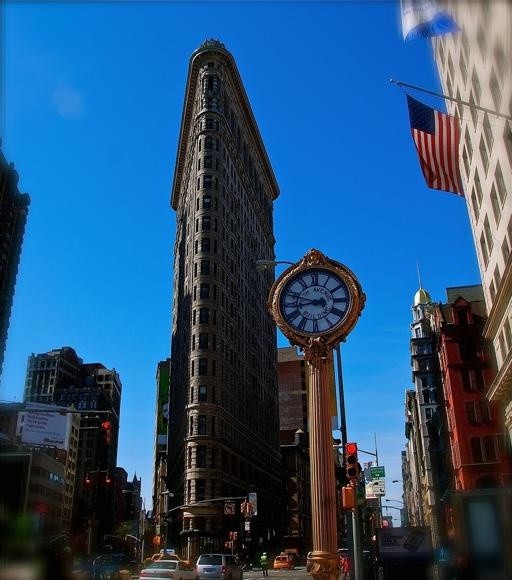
[397,0,461,45]
[403,89,467,201]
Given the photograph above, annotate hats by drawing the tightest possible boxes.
[263,552,267,555]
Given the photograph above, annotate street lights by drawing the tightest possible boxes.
[333,445,384,528]
[256,258,365,580]
[381,479,424,526]
[86,465,111,564]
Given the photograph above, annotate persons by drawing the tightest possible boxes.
[338,553,353,580]
[258,552,270,578]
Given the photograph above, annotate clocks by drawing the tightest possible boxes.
[264,248,366,370]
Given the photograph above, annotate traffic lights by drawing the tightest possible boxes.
[101,422,111,446]
[344,442,360,478]
[342,486,355,509]
[241,502,246,514]
[247,503,252,514]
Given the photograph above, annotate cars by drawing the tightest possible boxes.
[89,547,373,580]
